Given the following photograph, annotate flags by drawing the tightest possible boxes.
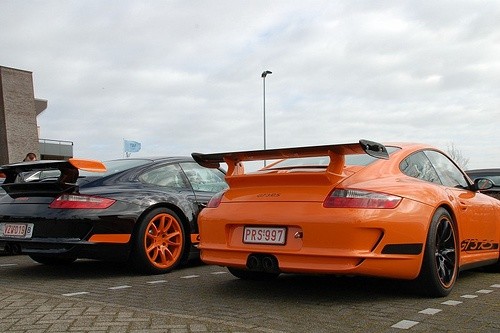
[124,140,141,152]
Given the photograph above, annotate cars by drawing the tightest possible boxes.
[463,168,500,201]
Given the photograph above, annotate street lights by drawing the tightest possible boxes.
[261,71,273,168]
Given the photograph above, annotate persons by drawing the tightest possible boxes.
[22,153,37,162]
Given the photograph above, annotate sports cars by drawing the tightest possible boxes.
[0,153,231,274]
[190,139,500,298]
